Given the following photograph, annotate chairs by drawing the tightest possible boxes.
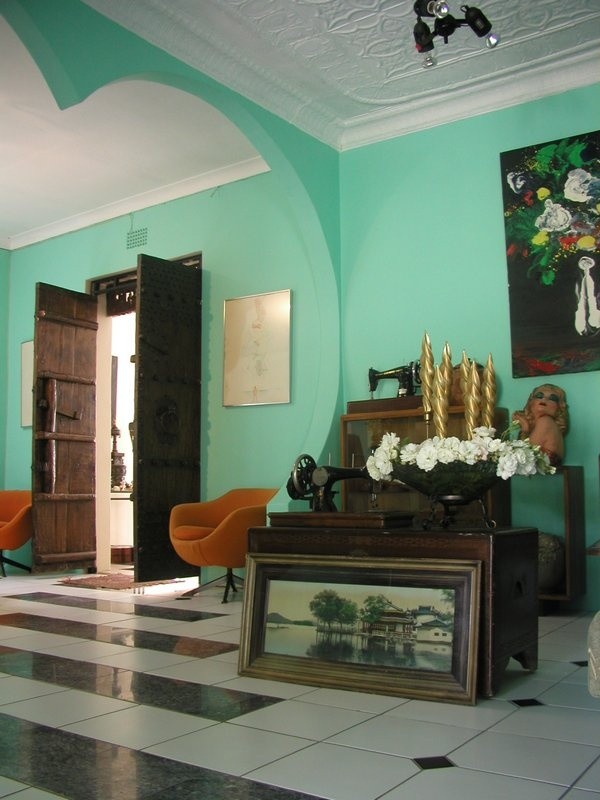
[170,488,279,604]
[0,489,34,576]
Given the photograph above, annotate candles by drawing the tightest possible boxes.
[419,330,452,438]
[460,350,496,440]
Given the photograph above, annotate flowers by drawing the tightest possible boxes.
[366,420,556,484]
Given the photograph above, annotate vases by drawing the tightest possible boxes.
[391,461,503,534]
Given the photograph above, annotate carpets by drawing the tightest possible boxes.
[56,573,186,592]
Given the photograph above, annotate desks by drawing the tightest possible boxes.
[244,512,540,697]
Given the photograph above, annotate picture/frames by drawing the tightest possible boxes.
[222,288,292,407]
[21,340,34,426]
[239,553,482,707]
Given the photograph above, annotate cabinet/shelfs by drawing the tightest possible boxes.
[339,405,587,612]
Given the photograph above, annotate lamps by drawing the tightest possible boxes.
[414,0,501,69]
